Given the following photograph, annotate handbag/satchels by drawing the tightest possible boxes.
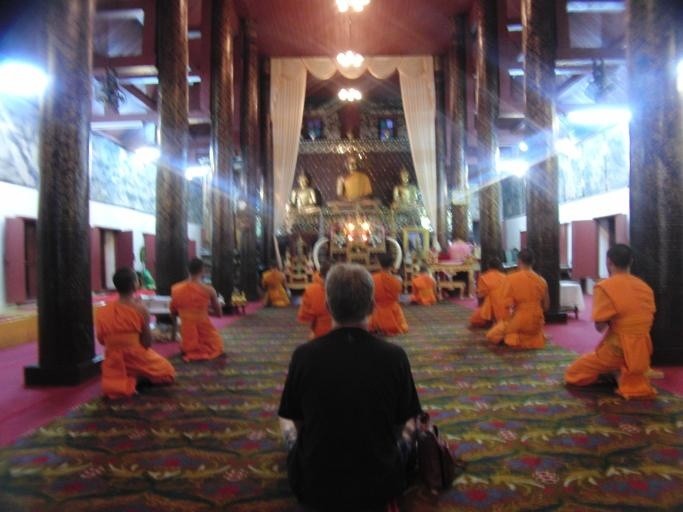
[414,411,455,490]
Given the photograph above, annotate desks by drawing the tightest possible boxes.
[148,308,182,341]
[560,282,586,320]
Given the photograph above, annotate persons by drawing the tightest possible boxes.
[369,251,408,334]
[325,154,379,211]
[388,164,420,210]
[567,243,658,398]
[261,264,292,307]
[438,236,470,297]
[297,261,336,341]
[286,169,321,214]
[411,265,437,306]
[275,261,422,512]
[467,249,549,348]
[96,266,176,398]
[169,253,223,361]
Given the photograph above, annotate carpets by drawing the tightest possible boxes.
[2,295,683,512]
